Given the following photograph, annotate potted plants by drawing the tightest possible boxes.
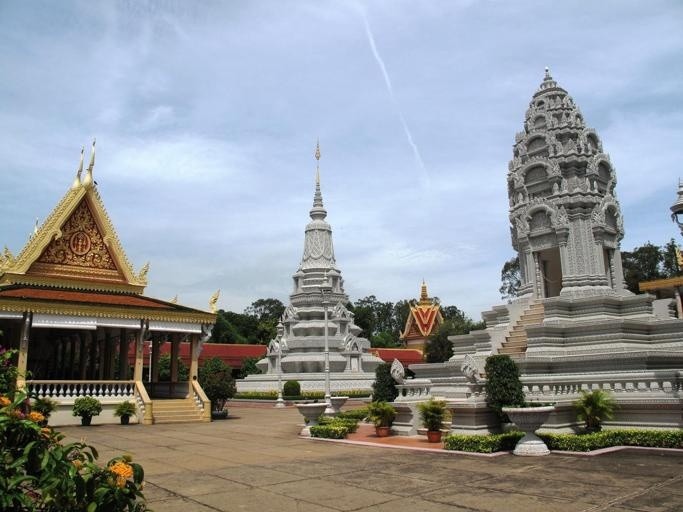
[294,392,349,436]
[71,395,102,426]
[112,400,136,425]
[202,371,236,420]
[30,397,59,428]
[370,400,398,437]
[503,400,554,458]
[415,398,450,442]
[574,389,622,436]
